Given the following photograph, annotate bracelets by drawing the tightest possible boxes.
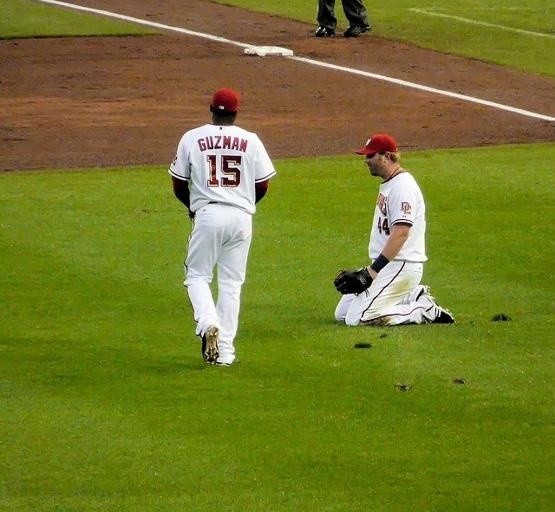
[369,254,389,274]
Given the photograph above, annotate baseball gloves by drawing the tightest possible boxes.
[334,266,372,295]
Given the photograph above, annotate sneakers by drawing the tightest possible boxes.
[416,285,454,323]
[201,326,231,366]
[344,24,371,37]
[316,26,335,36]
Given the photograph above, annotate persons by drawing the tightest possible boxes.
[315,0,371,38]
[167,88,277,368]
[332,133,455,327]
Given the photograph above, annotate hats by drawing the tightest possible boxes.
[355,134,397,155]
[208,88,240,113]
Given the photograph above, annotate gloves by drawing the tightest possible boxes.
[335,268,372,294]
